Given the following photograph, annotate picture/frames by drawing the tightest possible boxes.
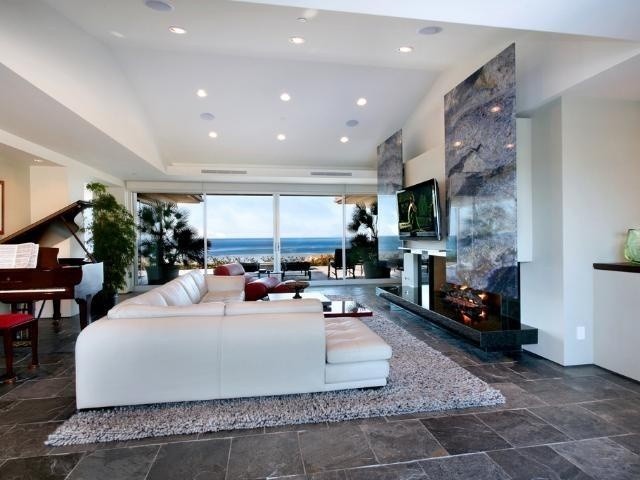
[0,180,4,236]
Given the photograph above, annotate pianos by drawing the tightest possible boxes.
[1,200,104,342]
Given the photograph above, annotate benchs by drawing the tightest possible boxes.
[281,259,312,281]
[240,263,260,279]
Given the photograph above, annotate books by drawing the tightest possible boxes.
[0,241,41,270]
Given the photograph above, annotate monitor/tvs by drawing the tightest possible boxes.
[395,177,443,242]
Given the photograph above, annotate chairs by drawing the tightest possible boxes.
[213,262,296,301]
[328,248,356,279]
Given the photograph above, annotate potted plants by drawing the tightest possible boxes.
[77,180,137,323]
[345,199,390,278]
[137,198,211,284]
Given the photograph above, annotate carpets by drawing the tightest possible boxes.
[44,294,506,447]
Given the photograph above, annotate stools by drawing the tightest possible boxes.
[0,313,40,386]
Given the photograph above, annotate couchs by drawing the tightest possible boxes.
[75,269,392,411]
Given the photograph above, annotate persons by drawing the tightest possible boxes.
[407,191,420,229]
